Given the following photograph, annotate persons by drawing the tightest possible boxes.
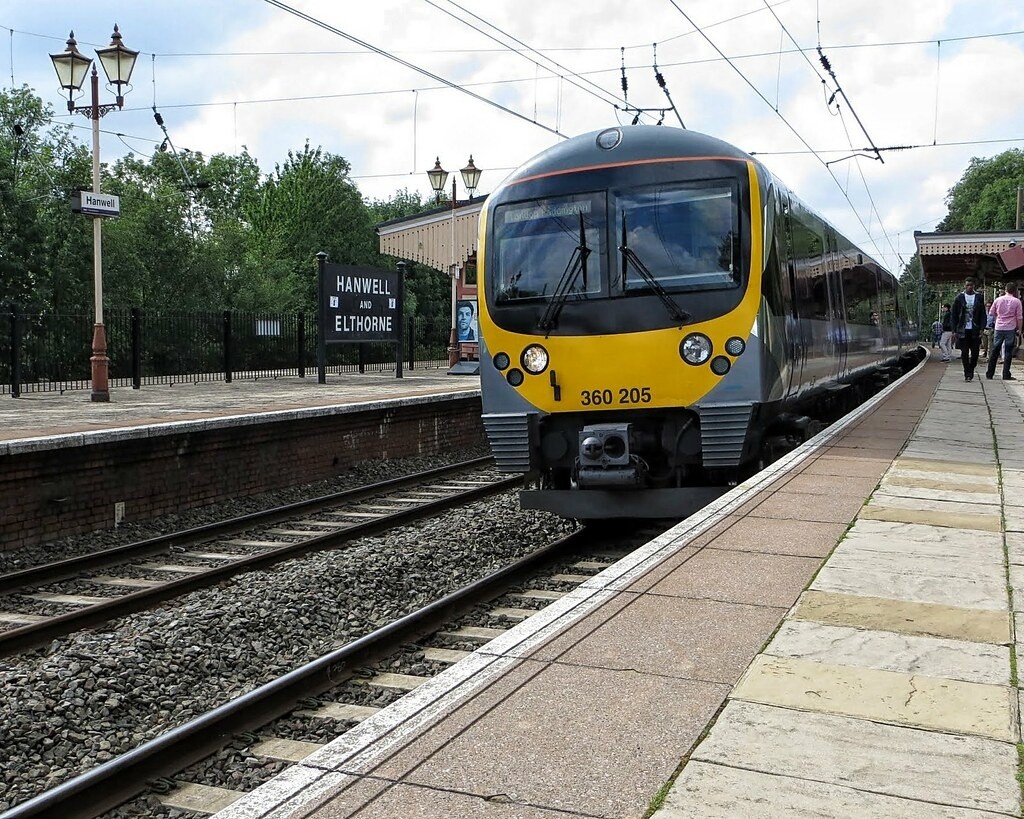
[932,318,943,348]
[986,282,1022,380]
[456,301,474,340]
[940,303,954,363]
[982,301,996,363]
[869,312,879,327]
[950,277,987,382]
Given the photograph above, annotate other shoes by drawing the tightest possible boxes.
[983,358,989,363]
[965,377,971,381]
[988,376,993,379]
[1003,376,1016,380]
[940,359,949,363]
[981,352,986,358]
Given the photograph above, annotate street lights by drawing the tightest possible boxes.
[426,151,483,370]
[46,19,142,406]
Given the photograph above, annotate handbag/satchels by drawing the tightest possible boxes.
[955,337,961,349]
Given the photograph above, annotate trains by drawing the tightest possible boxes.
[479,123,919,543]
